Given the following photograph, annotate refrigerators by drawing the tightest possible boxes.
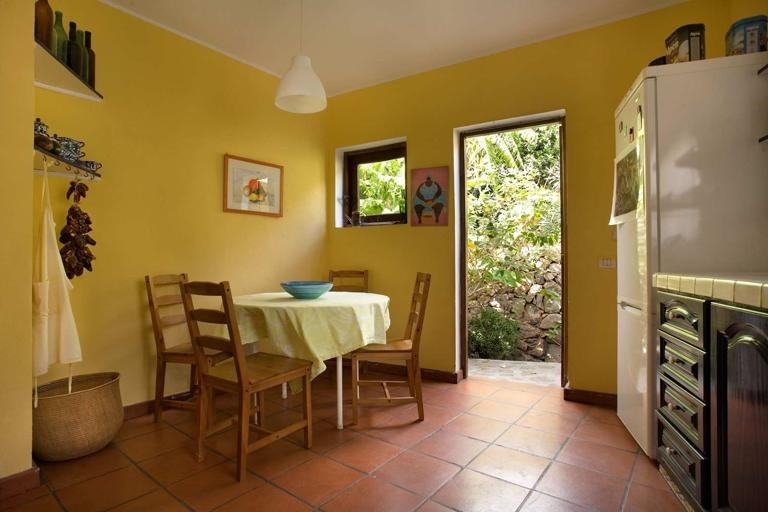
[615,51,768,461]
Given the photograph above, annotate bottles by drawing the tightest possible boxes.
[35,0,95,92]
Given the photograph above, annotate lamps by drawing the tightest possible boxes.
[274,0,327,115]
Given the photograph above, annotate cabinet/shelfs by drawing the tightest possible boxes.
[650,289,709,510]
[709,301,768,512]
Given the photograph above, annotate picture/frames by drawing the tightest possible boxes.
[222,153,285,219]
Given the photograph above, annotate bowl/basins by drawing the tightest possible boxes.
[280,281,334,299]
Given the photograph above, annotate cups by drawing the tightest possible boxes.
[35,119,102,173]
[351,210,365,227]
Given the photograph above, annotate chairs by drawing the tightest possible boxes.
[146,274,231,425]
[349,271,433,427]
[176,280,315,484]
[328,267,370,293]
